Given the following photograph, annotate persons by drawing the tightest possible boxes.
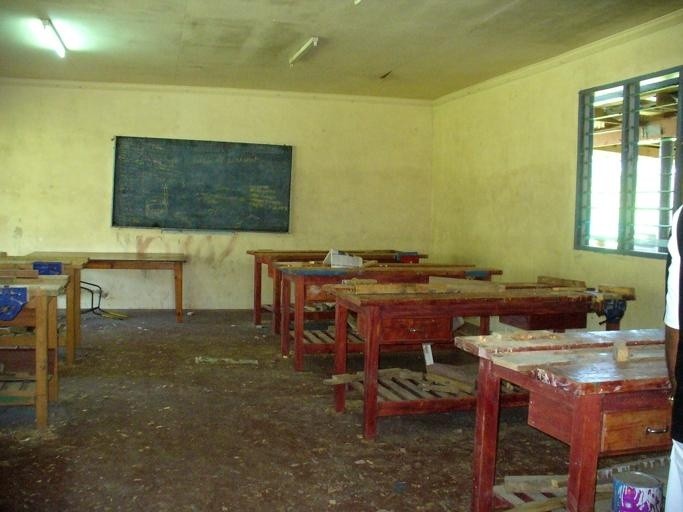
[662,202,683,512]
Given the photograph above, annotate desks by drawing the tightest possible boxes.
[0,252,186,428]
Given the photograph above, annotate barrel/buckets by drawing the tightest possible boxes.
[612,471,664,512]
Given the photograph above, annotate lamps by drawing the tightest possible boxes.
[41,18,66,58]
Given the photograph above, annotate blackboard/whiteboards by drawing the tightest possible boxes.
[111,136,292,232]
